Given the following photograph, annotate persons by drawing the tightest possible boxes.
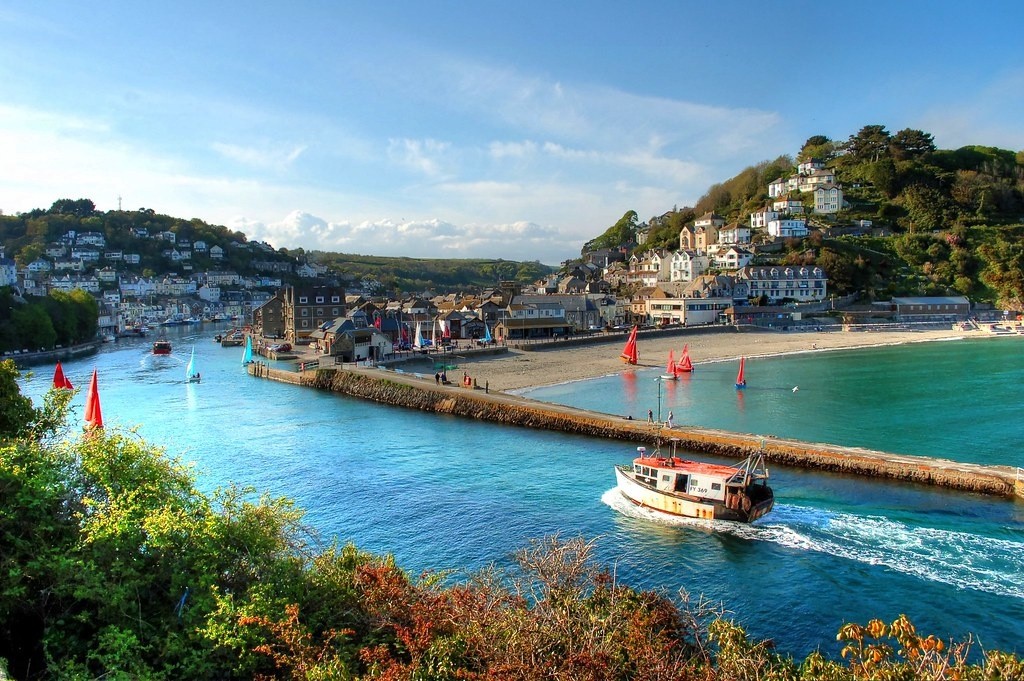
[434,373,447,385]
[668,411,674,428]
[196,373,200,378]
[553,333,558,341]
[647,410,655,426]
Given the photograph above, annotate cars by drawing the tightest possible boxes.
[274,343,292,352]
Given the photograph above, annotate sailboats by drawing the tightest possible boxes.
[393,313,437,352]
[676,343,694,372]
[734,357,747,389]
[186,346,201,380]
[53,359,74,390]
[242,335,255,366]
[619,325,638,365]
[660,349,679,380]
[83,369,104,432]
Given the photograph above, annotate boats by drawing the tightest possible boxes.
[133,317,200,337]
[215,313,228,322]
[615,429,774,523]
[103,335,116,342]
[153,329,171,354]
